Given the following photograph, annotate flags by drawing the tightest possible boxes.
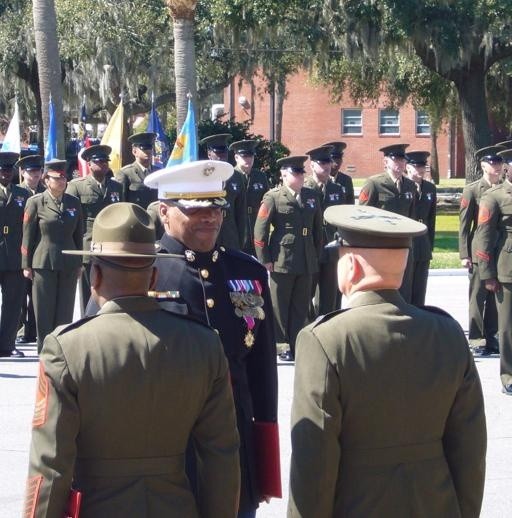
[76,103,91,179]
[145,101,171,172]
[43,97,57,165]
[0,102,21,154]
[98,96,123,183]
[164,100,198,169]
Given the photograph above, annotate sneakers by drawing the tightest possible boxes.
[42,158,70,179]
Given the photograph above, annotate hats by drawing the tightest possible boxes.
[405,149,431,168]
[0,152,20,170]
[140,159,237,210]
[61,201,187,271]
[304,144,336,164]
[322,141,347,162]
[473,140,512,165]
[198,132,233,154]
[276,155,309,175]
[228,139,258,155]
[14,154,42,172]
[126,131,157,150]
[322,203,430,252]
[379,143,410,160]
[80,144,111,162]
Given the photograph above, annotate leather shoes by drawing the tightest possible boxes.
[17,335,36,342]
[279,350,294,362]
[474,340,500,356]
[9,348,24,358]
[502,384,511,395]
